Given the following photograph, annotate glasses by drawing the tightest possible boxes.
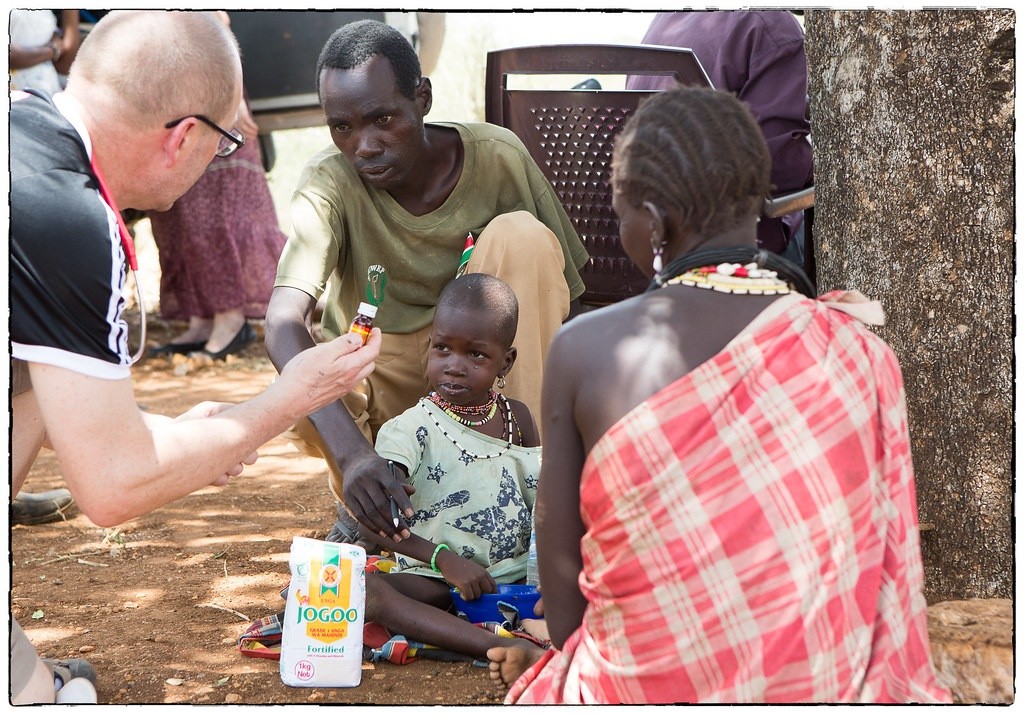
[164,113,245,158]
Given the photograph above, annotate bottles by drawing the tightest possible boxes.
[348,301,378,348]
[525,500,540,585]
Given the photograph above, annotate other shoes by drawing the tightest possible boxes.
[146,338,210,359]
[186,316,258,364]
[44,657,98,698]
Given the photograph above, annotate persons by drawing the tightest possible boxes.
[10,8,81,99]
[366,271,543,698]
[6,8,383,705]
[10,488,76,528]
[619,8,819,299]
[264,18,589,554]
[494,87,946,705]
[147,88,290,361]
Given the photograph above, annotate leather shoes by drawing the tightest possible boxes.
[10,488,75,527]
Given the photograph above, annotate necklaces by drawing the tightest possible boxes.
[419,391,523,460]
[646,248,816,297]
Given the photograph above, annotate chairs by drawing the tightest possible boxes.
[484,43,716,312]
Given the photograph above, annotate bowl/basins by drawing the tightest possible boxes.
[449,584,545,625]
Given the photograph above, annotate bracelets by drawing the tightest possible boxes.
[46,44,61,62]
[431,543,450,573]
